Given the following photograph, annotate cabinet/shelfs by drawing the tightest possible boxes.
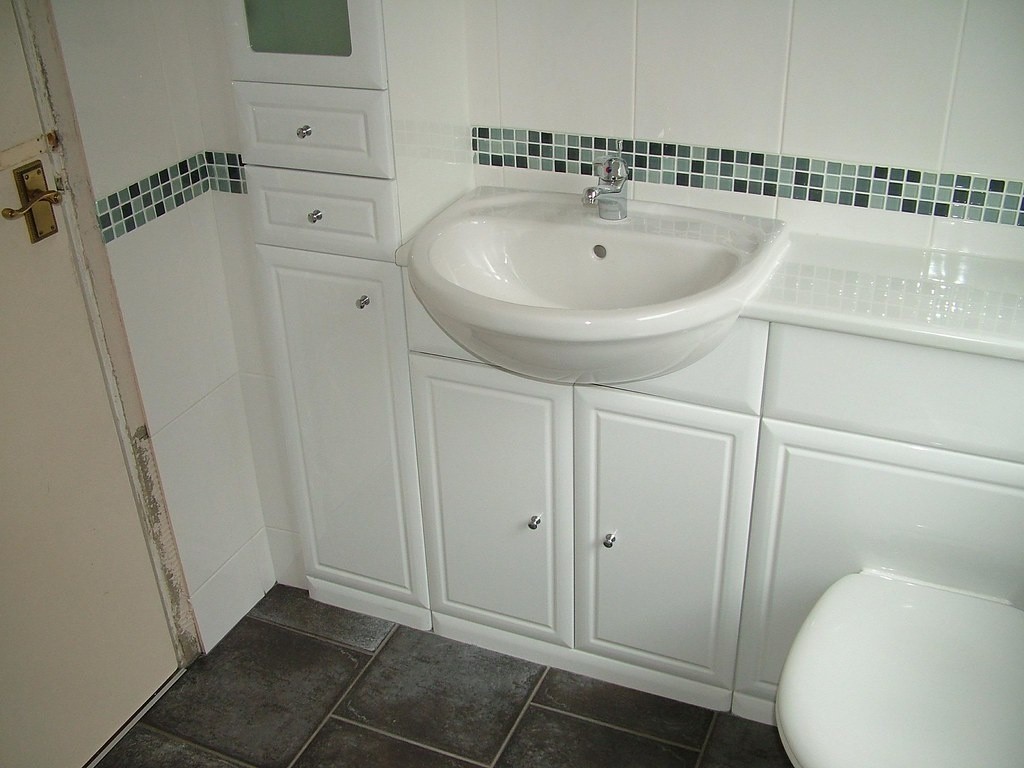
[227,1,433,635]
[734,310,1024,737]
[401,265,769,715]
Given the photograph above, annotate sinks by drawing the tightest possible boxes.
[397,185,795,388]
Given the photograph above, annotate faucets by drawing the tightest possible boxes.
[583,152,631,221]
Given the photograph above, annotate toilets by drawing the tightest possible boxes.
[772,559,1023,768]
